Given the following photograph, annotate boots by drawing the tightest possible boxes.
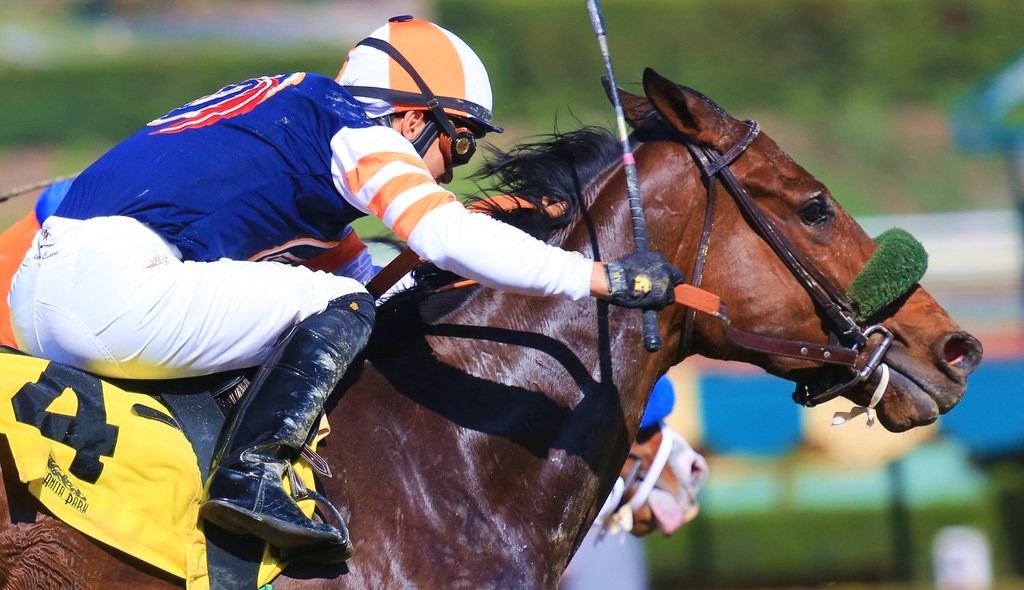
[199,325,355,563]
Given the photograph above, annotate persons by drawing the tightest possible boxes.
[1,12,686,590]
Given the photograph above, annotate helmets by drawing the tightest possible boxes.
[335,15,504,133]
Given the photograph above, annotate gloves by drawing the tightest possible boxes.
[603,252,686,311]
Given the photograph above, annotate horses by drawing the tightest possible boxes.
[0,66,986,588]
